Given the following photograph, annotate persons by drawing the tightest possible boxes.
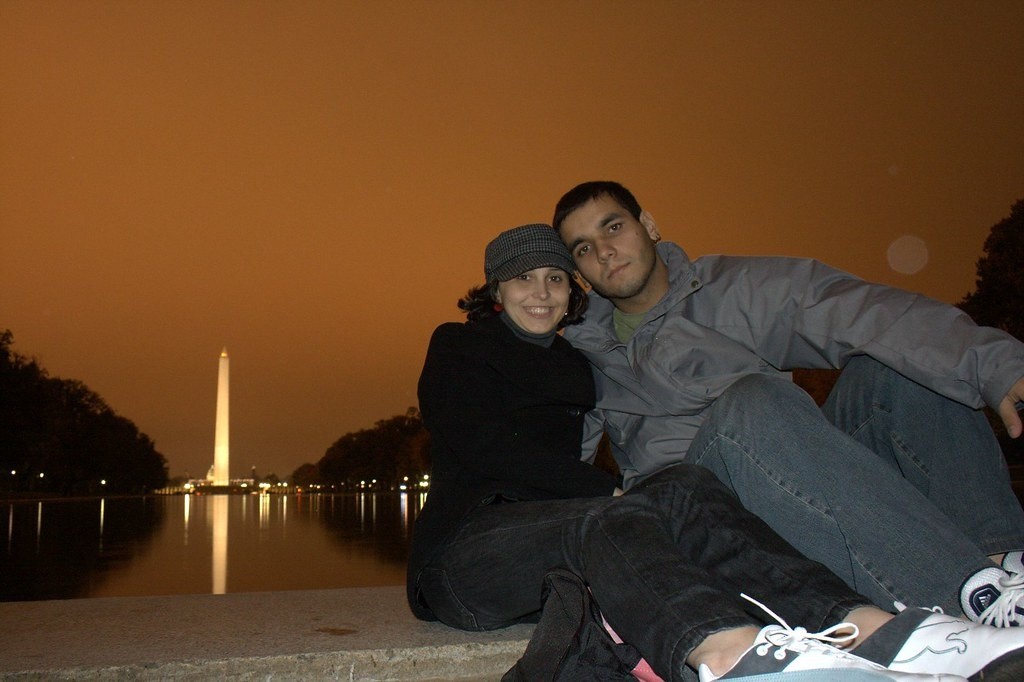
[405,224,1024,681]
[552,180,1024,626]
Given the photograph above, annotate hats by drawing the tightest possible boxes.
[484,223,576,285]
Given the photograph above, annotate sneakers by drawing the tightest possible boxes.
[698,593,888,682]
[848,600,1024,682]
[959,550,1024,628]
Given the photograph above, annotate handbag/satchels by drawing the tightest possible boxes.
[502,568,640,681]
